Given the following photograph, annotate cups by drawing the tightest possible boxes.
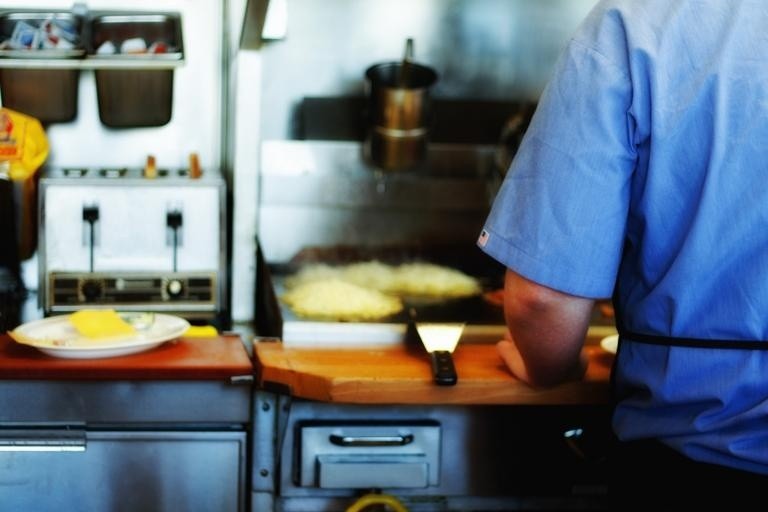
[361,61,439,170]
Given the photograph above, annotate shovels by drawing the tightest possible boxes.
[413,319,468,385]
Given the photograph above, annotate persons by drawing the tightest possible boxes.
[477,0,768,512]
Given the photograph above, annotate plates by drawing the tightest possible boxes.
[600,334,620,357]
[10,310,190,359]
